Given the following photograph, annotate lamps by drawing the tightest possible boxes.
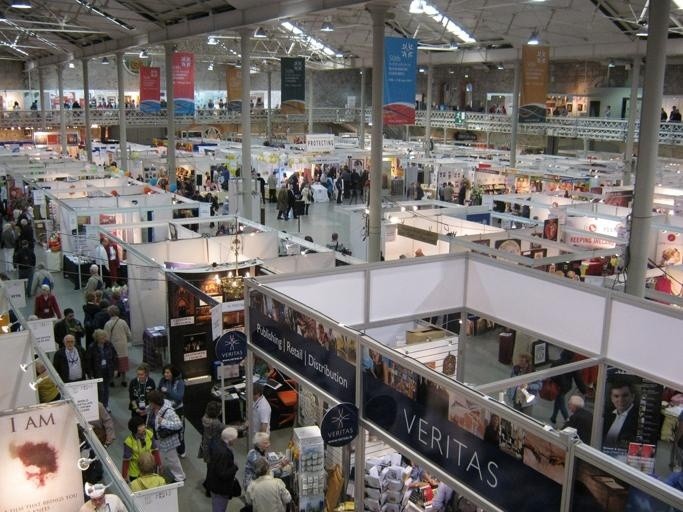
[527,32,539,45]
[215,217,244,300]
[498,385,535,407]
[101,21,333,71]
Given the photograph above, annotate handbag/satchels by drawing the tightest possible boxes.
[86,350,107,380]
[94,277,103,290]
[39,272,50,285]
[539,379,559,402]
[230,479,241,499]
[307,192,311,201]
[158,425,184,439]
[198,444,203,458]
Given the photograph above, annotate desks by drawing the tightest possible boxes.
[309,184,329,203]
[63,254,95,290]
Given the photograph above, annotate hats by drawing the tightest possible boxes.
[84,481,105,498]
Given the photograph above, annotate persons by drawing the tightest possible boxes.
[553,106,568,125]
[255,172,314,221]
[354,159,363,175]
[30,239,131,511]
[160,99,167,116]
[604,105,611,126]
[279,229,352,269]
[123,361,187,492]
[9,98,136,130]
[197,98,234,119]
[66,153,118,178]
[407,182,423,210]
[1,174,34,298]
[439,181,468,205]
[607,254,625,276]
[249,97,264,116]
[198,360,293,511]
[659,106,681,138]
[400,454,454,511]
[416,99,508,122]
[318,166,370,205]
[137,169,229,231]
[603,380,639,449]
[507,349,594,448]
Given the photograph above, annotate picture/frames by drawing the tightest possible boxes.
[532,340,548,366]
